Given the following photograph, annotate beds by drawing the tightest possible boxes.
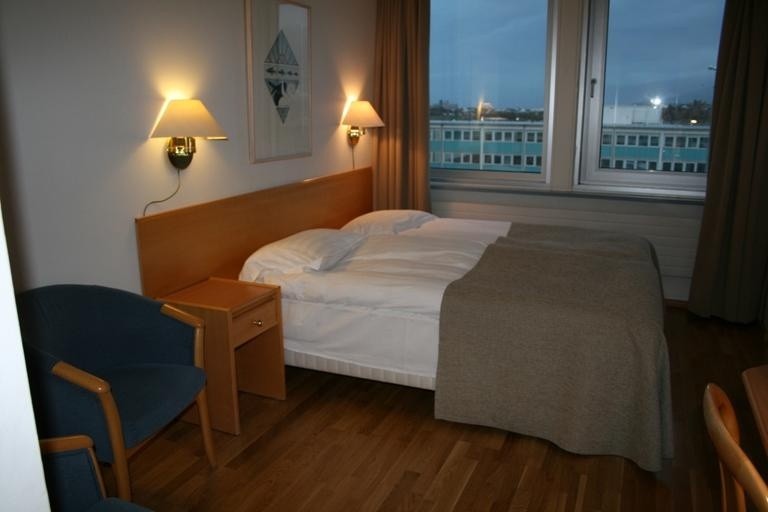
[134,166,675,479]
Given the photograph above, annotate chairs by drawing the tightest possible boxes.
[43,436,155,511]
[700,381,767,510]
[15,283,218,503]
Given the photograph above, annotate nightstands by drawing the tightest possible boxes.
[155,276,287,436]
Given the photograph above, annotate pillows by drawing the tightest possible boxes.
[245,226,362,271]
[341,207,434,240]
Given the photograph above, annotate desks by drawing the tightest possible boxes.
[741,364,768,450]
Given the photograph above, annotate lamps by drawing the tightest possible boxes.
[341,99,386,147]
[151,99,230,168]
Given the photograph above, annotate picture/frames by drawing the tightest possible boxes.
[245,0,314,165]
